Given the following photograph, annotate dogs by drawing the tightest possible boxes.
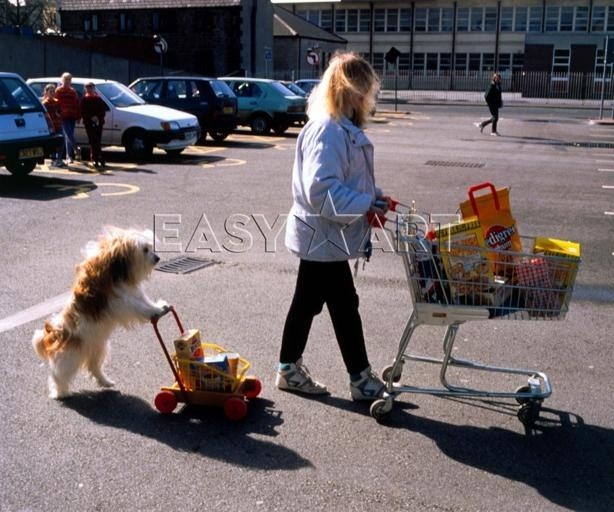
[32,225,171,399]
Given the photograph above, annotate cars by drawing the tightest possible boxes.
[0,72,56,177]
[10,77,198,162]
[216,77,307,135]
[127,77,240,145]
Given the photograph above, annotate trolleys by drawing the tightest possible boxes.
[151,306,262,420]
[365,198,583,426]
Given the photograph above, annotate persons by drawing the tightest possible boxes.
[276,53,403,402]
[41,84,63,167]
[54,73,80,164]
[476,74,504,137]
[81,82,106,168]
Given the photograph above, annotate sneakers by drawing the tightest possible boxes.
[276,359,327,396]
[476,122,484,133]
[50,162,56,170]
[74,148,81,162]
[65,158,73,165]
[92,160,98,167]
[101,161,106,168]
[348,367,403,402]
[56,162,69,169]
[491,132,501,137]
